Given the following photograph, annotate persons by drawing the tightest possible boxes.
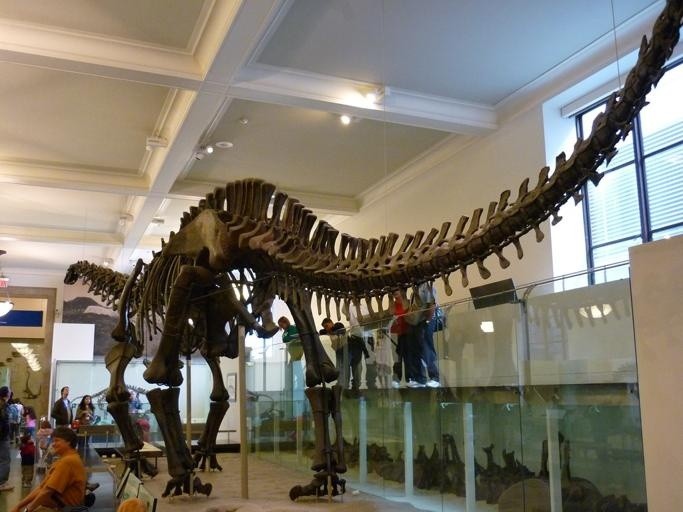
[0,386,14,490]
[278,316,305,390]
[51,387,73,428]
[8,426,87,512]
[118,498,147,512]
[406,281,441,388]
[19,431,37,488]
[389,289,415,389]
[318,318,350,391]
[348,301,377,391]
[7,398,37,448]
[76,395,95,420]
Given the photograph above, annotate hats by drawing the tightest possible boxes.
[41,421,51,428]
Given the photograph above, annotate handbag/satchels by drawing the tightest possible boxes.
[64,505,89,512]
[404,305,422,326]
[434,315,445,332]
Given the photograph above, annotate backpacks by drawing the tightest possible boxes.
[8,404,19,422]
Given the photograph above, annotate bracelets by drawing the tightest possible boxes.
[22,508,28,512]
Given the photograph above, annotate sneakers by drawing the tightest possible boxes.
[15,438,20,444]
[350,384,390,391]
[405,380,426,388]
[1,482,32,491]
[391,381,400,390]
[10,442,14,444]
[425,380,440,388]
